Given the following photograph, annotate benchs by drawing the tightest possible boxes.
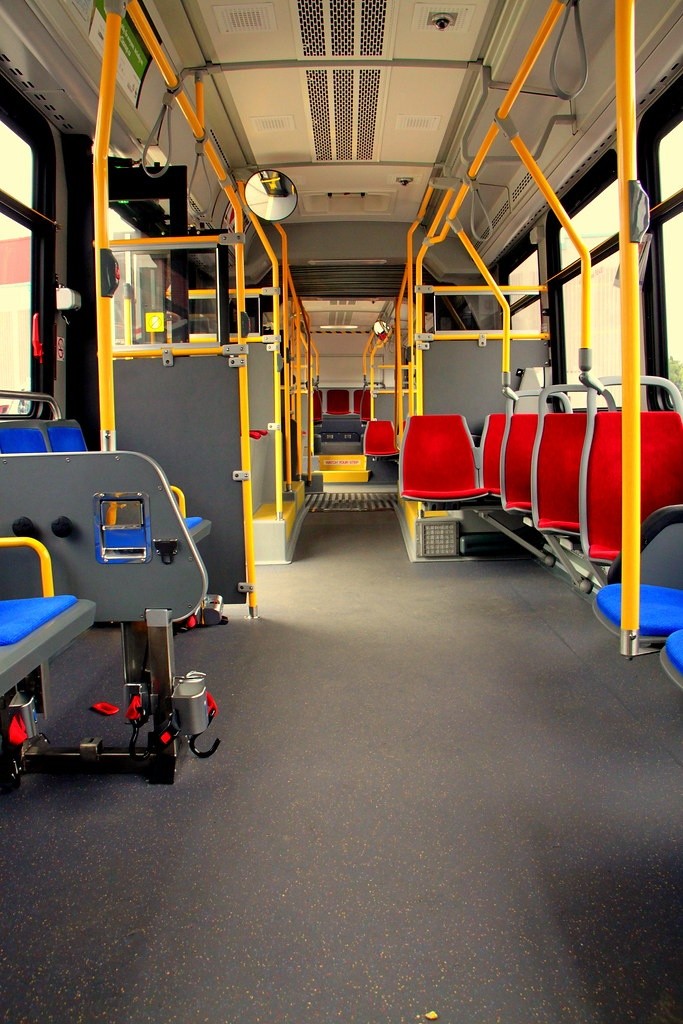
[0,390,213,687]
[0,538,95,700]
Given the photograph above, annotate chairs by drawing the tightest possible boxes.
[499,389,573,551]
[578,376,683,564]
[326,389,351,415]
[353,390,363,414]
[659,630,683,690]
[530,384,618,593]
[363,421,399,461]
[400,414,488,502]
[313,390,323,420]
[362,390,371,421]
[593,503,683,662]
[477,413,515,497]
